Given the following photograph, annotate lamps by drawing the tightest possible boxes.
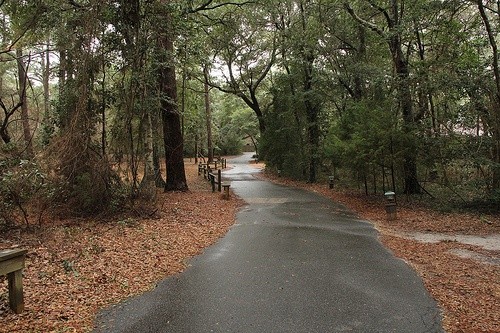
[385,190,396,203]
[278,168,281,174]
[328,173,334,183]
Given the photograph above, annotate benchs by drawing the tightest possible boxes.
[0,248,28,315]
